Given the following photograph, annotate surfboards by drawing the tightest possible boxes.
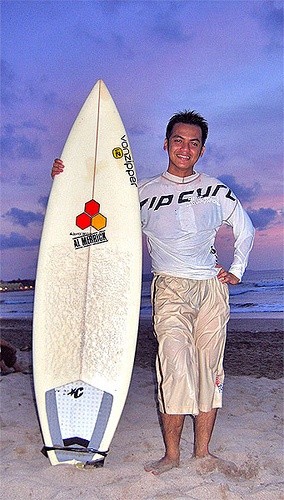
[31,79,142,469]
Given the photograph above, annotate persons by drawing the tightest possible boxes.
[49,109,255,476]
